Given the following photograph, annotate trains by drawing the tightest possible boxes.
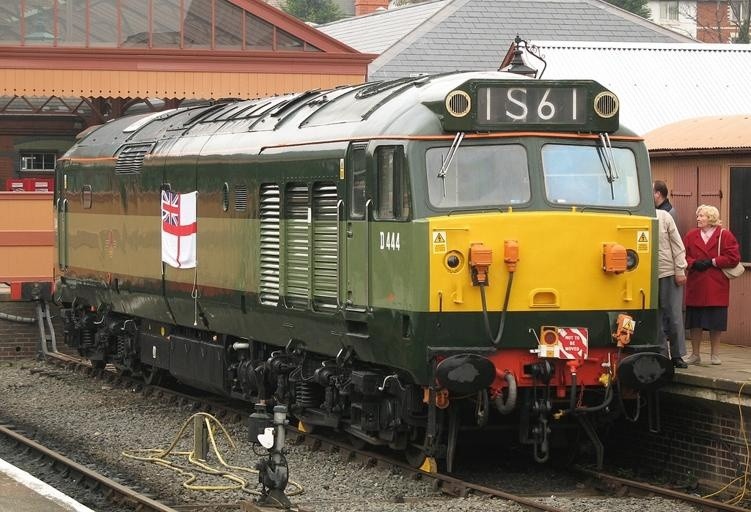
[33,67,678,478]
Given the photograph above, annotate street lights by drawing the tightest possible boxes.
[506,35,547,80]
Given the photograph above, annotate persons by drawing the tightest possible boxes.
[682,205,741,365]
[652,179,683,240]
[655,208,689,368]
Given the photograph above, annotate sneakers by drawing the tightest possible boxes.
[671,357,688,368]
[684,352,700,365]
[711,354,721,364]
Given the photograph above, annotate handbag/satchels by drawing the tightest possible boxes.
[720,260,745,279]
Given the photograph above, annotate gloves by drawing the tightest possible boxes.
[693,260,712,271]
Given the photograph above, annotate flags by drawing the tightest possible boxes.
[162,189,197,268]
